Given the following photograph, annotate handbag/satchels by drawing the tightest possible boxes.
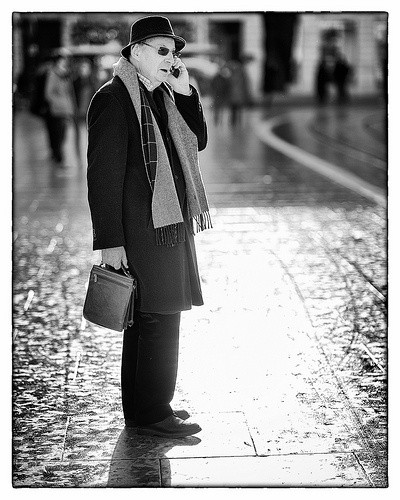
[83,261,137,332]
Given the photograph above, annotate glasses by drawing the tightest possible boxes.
[139,41,182,59]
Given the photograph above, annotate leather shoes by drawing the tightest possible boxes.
[125,409,190,430]
[137,412,203,437]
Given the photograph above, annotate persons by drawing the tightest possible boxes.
[13,26,387,166]
[86,16,213,441]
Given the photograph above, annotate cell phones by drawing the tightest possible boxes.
[169,65,180,79]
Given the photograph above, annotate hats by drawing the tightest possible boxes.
[120,15,187,61]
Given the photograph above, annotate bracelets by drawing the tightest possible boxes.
[184,87,192,95]
